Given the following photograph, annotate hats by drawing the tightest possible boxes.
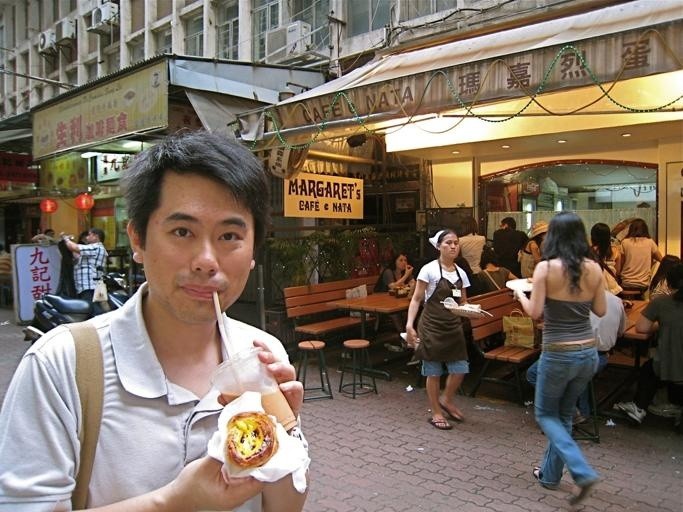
[429,230,445,250]
[531,220,549,238]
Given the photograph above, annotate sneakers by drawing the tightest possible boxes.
[388,345,403,353]
[571,407,586,426]
[406,354,423,365]
[648,403,681,419]
[617,400,648,424]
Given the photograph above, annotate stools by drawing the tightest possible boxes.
[543,352,602,444]
[296,340,334,403]
[339,340,378,398]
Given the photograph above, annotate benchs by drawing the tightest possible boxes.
[276,273,380,366]
[463,287,541,405]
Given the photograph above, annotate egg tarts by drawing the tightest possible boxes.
[225,412,279,469]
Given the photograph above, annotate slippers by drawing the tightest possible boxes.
[438,400,465,421]
[427,417,453,430]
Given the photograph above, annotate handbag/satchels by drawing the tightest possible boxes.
[502,309,535,351]
[346,284,370,318]
[591,246,625,291]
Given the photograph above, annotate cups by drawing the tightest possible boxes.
[209,346,298,433]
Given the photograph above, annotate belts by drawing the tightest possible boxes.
[541,339,597,352]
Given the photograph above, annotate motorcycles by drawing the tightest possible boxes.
[22,265,131,344]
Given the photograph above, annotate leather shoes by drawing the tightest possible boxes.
[569,478,600,507]
[533,465,556,491]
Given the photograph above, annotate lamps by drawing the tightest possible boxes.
[81,152,103,159]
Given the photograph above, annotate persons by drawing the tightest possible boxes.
[493,217,530,279]
[456,216,486,275]
[60,228,107,316]
[405,229,488,430]
[371,250,414,351]
[519,220,549,278]
[617,255,683,424]
[77,230,88,244]
[469,245,518,297]
[0,129,311,512]
[30,230,54,244]
[621,218,663,289]
[525,290,626,430]
[514,212,607,507]
[588,223,621,296]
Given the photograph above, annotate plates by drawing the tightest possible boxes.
[505,278,537,292]
